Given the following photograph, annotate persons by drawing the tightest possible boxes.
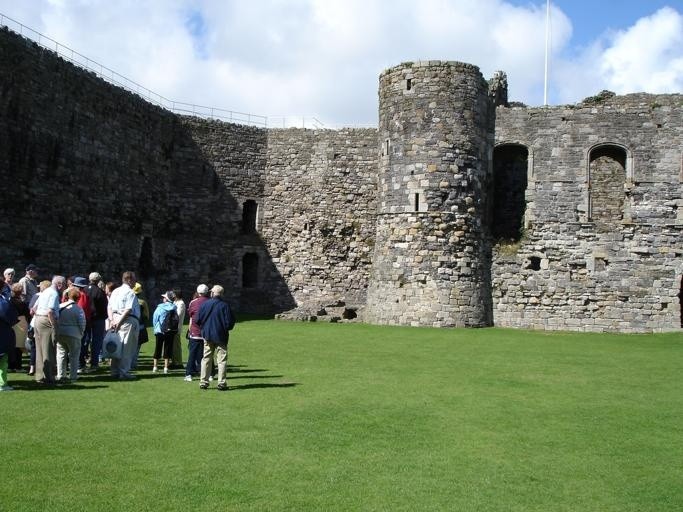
[151,290,177,374]
[168,287,186,369]
[183,283,215,382]
[0,263,150,390]
[194,284,235,391]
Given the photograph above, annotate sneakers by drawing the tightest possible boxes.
[0,385,13,390]
[153,364,183,373]
[74,366,100,379]
[8,368,72,387]
[183,375,228,390]
[112,373,135,380]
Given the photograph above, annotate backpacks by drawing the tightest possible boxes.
[158,310,179,338]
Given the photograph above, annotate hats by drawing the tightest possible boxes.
[89,272,102,280]
[209,284,224,293]
[160,291,174,303]
[133,282,142,294]
[72,277,88,288]
[197,284,209,294]
[25,264,41,270]
